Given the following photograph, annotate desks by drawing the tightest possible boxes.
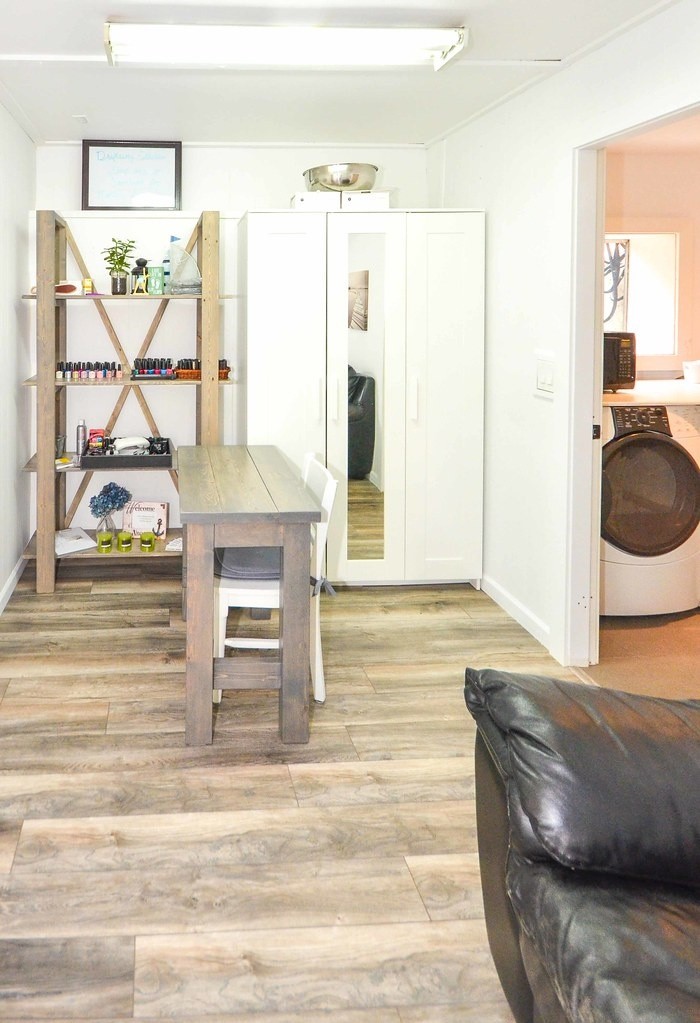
[463,666,700,1022]
[177,445,322,748]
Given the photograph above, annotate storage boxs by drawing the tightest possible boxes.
[122,501,170,539]
[148,267,164,295]
[175,367,231,379]
[79,437,176,470]
[289,189,389,210]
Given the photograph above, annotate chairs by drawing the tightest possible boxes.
[212,457,336,705]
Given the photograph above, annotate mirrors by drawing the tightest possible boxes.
[327,215,406,580]
[604,231,681,358]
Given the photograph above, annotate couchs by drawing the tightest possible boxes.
[348,364,375,480]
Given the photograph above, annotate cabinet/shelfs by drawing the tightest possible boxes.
[23,209,233,594]
[236,211,486,590]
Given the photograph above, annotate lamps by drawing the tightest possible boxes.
[102,22,471,73]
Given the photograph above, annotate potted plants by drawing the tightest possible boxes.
[102,237,136,295]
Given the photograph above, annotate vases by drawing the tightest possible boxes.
[95,515,116,542]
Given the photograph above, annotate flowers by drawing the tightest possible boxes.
[88,482,132,529]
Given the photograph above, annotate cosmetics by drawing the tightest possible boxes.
[76,420,86,455]
[55,357,227,379]
[85,428,172,456]
[96,531,156,553]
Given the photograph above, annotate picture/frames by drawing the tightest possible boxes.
[604,239,630,333]
[82,139,182,211]
